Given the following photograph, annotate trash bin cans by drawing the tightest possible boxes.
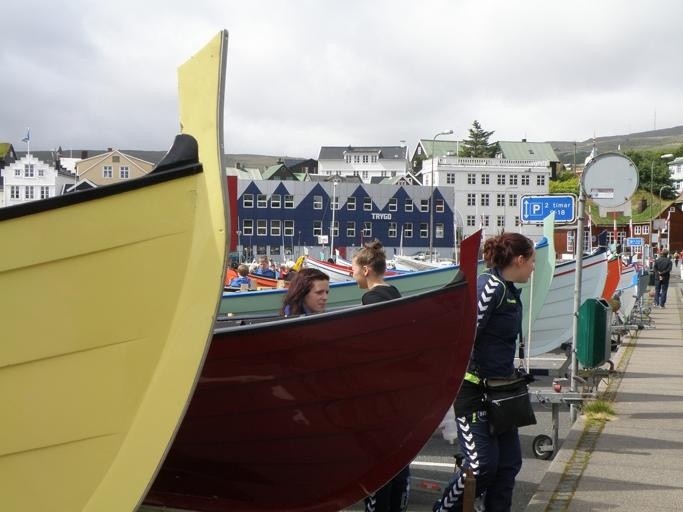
[577,298,612,368]
[649,274,654,285]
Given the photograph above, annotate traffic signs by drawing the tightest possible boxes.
[519,192,579,226]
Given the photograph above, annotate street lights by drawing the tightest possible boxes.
[648,153,674,270]
[429,130,454,264]
[330,181,339,259]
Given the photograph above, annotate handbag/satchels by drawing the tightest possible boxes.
[482,373,538,429]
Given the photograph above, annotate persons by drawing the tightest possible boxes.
[673,250,683,269]
[432,232,536,512]
[653,248,672,307]
[229,257,297,288]
[353,237,412,512]
[280,267,330,317]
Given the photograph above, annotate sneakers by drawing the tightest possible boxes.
[653,301,665,308]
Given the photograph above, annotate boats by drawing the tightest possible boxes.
[0,28,233,512]
